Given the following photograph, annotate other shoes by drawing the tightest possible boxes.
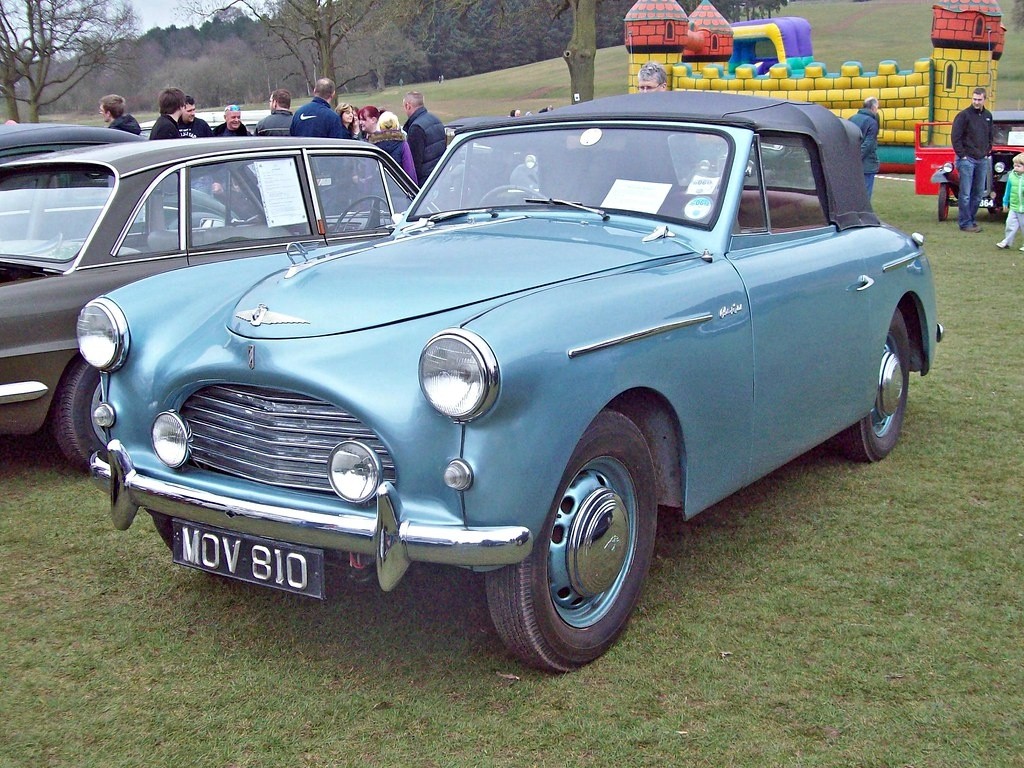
[1019,247,1024,251]
[962,223,981,232]
[996,242,1008,249]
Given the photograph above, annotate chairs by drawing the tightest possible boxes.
[146,231,180,253]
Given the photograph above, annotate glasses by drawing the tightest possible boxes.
[637,83,662,90]
[1013,165,1024,168]
[225,106,240,112]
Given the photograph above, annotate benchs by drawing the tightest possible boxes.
[670,190,828,228]
[191,225,294,245]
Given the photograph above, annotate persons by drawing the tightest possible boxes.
[439,74,444,84]
[99,93,141,136]
[508,105,553,117]
[289,76,351,215]
[847,95,880,202]
[212,105,253,136]
[332,102,418,198]
[148,87,186,140]
[399,78,403,88]
[252,88,295,136]
[637,61,668,94]
[401,89,446,200]
[176,95,225,201]
[996,150,1024,252]
[950,88,994,233]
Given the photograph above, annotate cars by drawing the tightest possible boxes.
[0,121,240,227]
[76,90,946,677]
[1,136,450,472]
[914,111,1024,222]
